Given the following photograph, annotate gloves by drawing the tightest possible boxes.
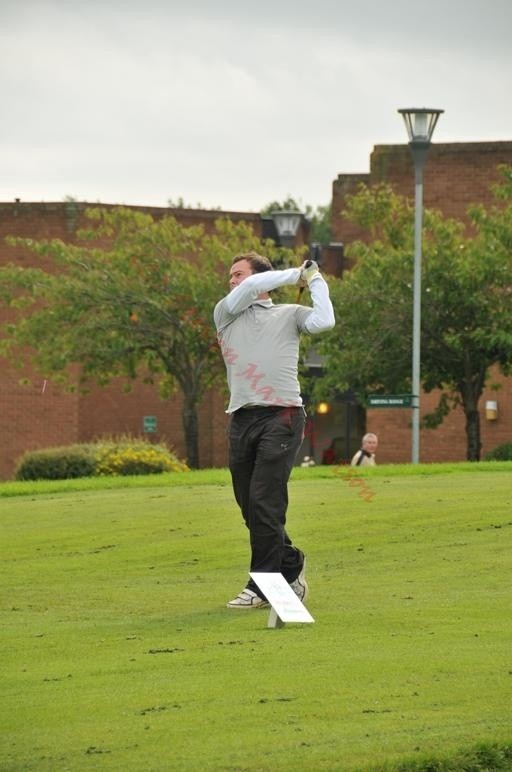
[301,260,318,280]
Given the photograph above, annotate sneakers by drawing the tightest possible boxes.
[289,556,308,603]
[226,588,271,609]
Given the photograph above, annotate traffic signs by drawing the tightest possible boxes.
[365,391,417,410]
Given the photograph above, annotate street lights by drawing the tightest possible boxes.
[398,107,445,463]
[270,208,306,249]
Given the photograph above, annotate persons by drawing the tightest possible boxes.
[350,433,378,466]
[214,250,336,610]
